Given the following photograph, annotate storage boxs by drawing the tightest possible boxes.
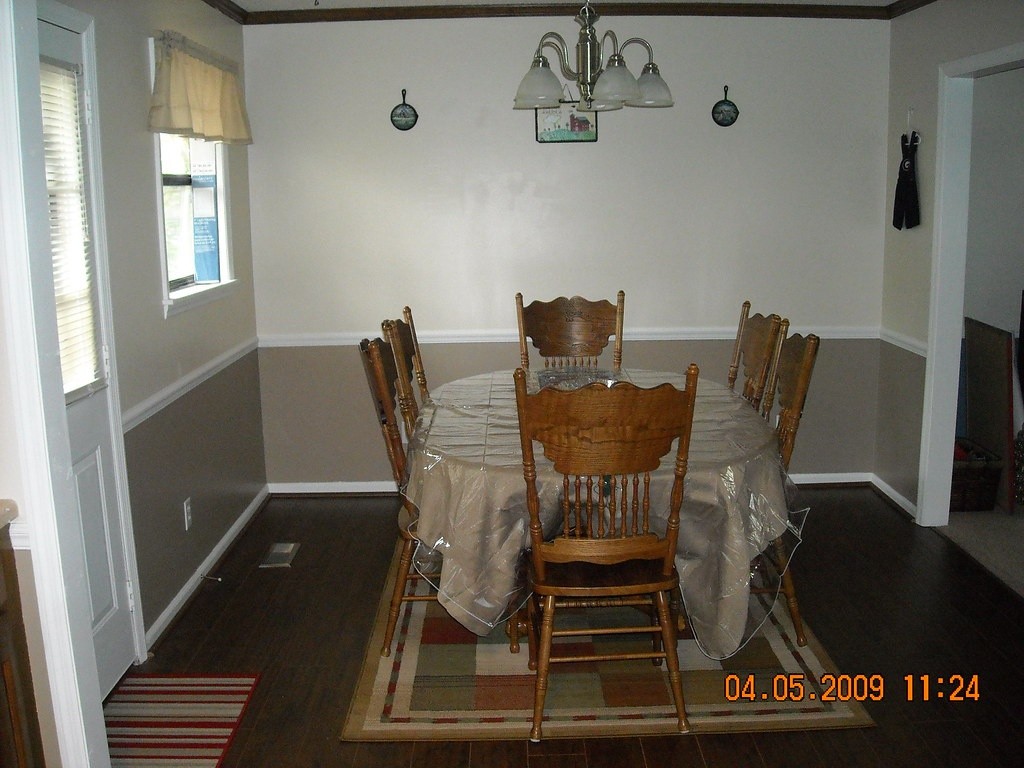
[950,438,1003,511]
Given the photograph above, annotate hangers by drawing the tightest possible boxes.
[902,105,921,146]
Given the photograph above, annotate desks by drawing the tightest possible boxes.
[400,367,808,660]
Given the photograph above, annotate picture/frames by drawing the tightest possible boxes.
[535,100,598,143]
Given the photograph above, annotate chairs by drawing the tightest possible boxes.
[512,291,699,743]
[359,304,523,657]
[728,301,820,647]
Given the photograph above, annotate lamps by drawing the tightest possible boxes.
[513,0,674,112]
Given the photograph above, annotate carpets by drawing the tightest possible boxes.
[105,668,258,768]
[341,539,877,742]
